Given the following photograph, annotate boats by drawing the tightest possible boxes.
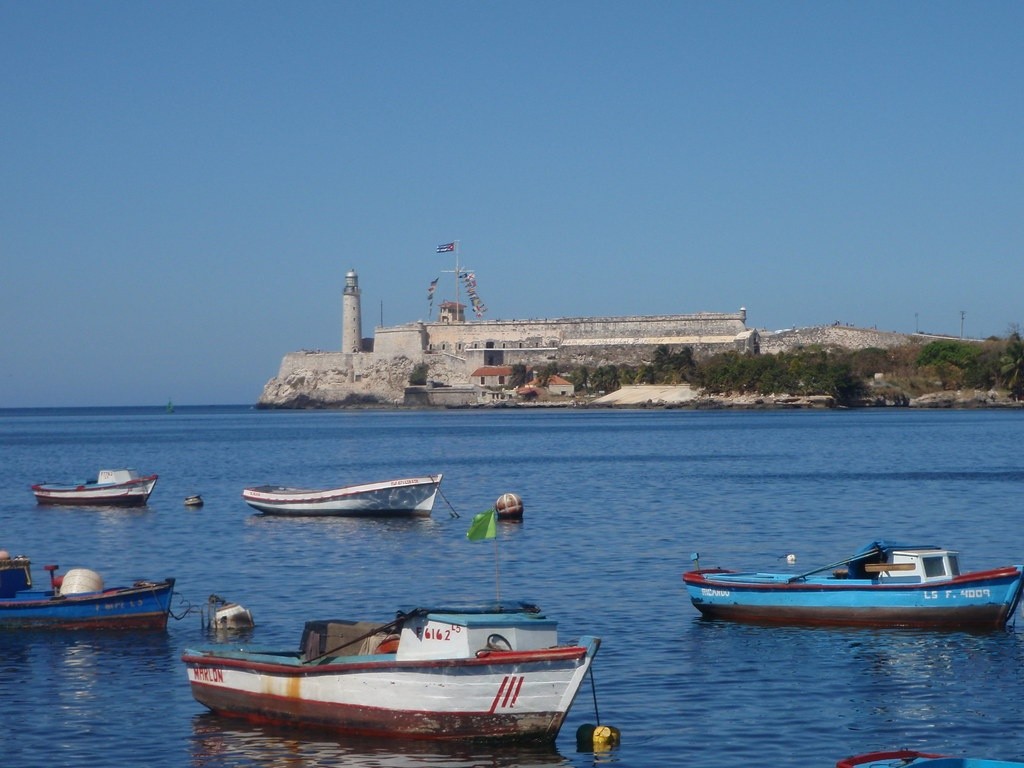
[0,550,176,633]
[182,600,602,747]
[32,470,159,506]
[241,473,443,518]
[837,746,1024,768]
[683,538,1023,630]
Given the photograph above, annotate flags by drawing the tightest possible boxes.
[428,277,439,306]
[436,242,454,252]
[458,272,487,317]
[467,511,495,540]
